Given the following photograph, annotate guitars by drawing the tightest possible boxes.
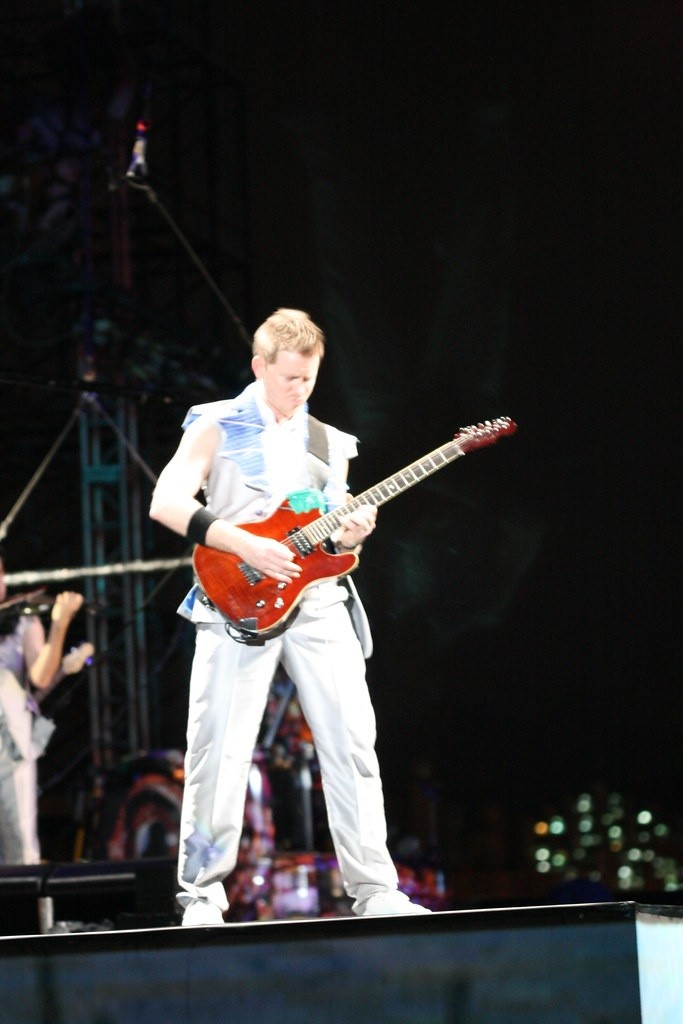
[191,413,519,638]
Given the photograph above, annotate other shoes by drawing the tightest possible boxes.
[181,898,225,926]
[362,894,431,916]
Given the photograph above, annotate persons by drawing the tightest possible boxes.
[148,309,436,929]
[1,565,94,866]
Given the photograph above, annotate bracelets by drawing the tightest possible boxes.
[186,505,222,545]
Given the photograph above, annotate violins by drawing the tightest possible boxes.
[0,589,112,620]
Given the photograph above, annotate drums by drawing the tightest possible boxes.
[92,677,445,921]
